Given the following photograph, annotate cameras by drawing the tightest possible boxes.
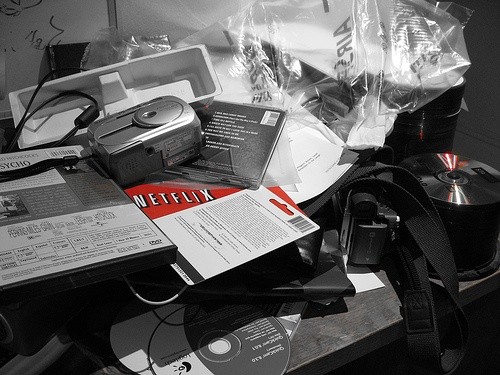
[334,170,399,267]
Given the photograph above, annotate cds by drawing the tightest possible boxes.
[146,303,292,375]
[401,152,499,209]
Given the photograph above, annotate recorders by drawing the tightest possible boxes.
[94,95,205,185]
